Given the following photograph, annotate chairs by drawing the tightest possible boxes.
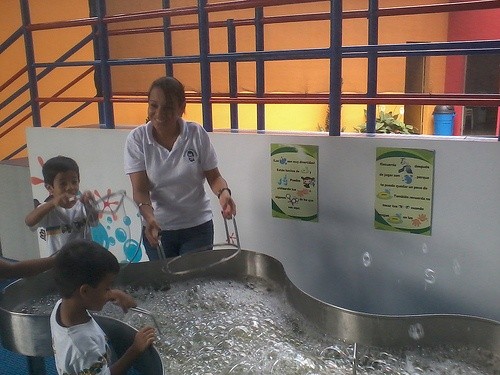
[463,106,474,131]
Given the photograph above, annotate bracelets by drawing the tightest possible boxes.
[218,187,232,200]
[139,202,154,212]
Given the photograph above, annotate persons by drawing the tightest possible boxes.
[123,76,236,261]
[0,256,53,278]
[48,240,158,375]
[25,156,98,260]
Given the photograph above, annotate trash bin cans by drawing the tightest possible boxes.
[432,104,456,136]
